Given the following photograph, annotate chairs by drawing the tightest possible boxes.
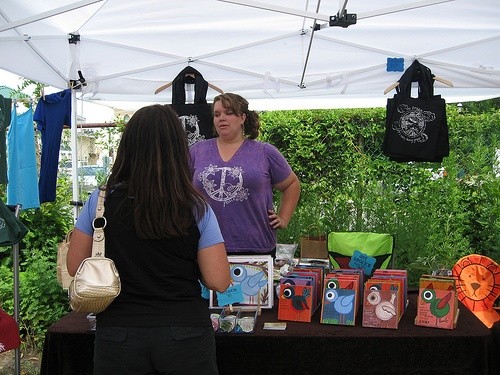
[326,231,395,283]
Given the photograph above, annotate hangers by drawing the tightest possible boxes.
[155,59,223,94]
[383,58,455,96]
[11,90,33,104]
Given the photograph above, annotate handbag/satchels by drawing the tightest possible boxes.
[382,60,449,165]
[56,229,74,291]
[67,190,122,313]
[301,224,329,259]
[167,66,220,148]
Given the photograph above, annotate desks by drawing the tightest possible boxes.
[39,292,500,375]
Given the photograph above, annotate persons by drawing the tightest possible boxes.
[187,92,300,264]
[65,103,232,375]
